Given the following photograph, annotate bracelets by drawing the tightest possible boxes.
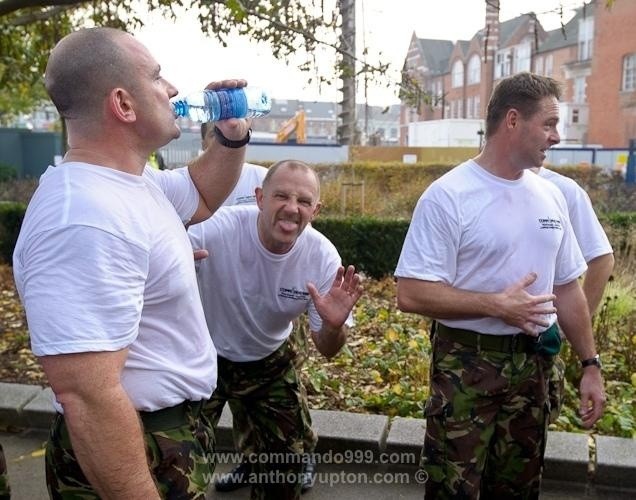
[212,124,252,150]
[579,352,602,370]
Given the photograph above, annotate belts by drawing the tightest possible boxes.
[435,322,543,355]
[48,397,202,441]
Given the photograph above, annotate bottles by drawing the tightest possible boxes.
[171,87,271,122]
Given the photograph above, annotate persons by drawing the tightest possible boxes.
[10,26,255,499]
[179,157,365,500]
[197,121,270,206]
[391,69,609,500]
[523,162,616,426]
